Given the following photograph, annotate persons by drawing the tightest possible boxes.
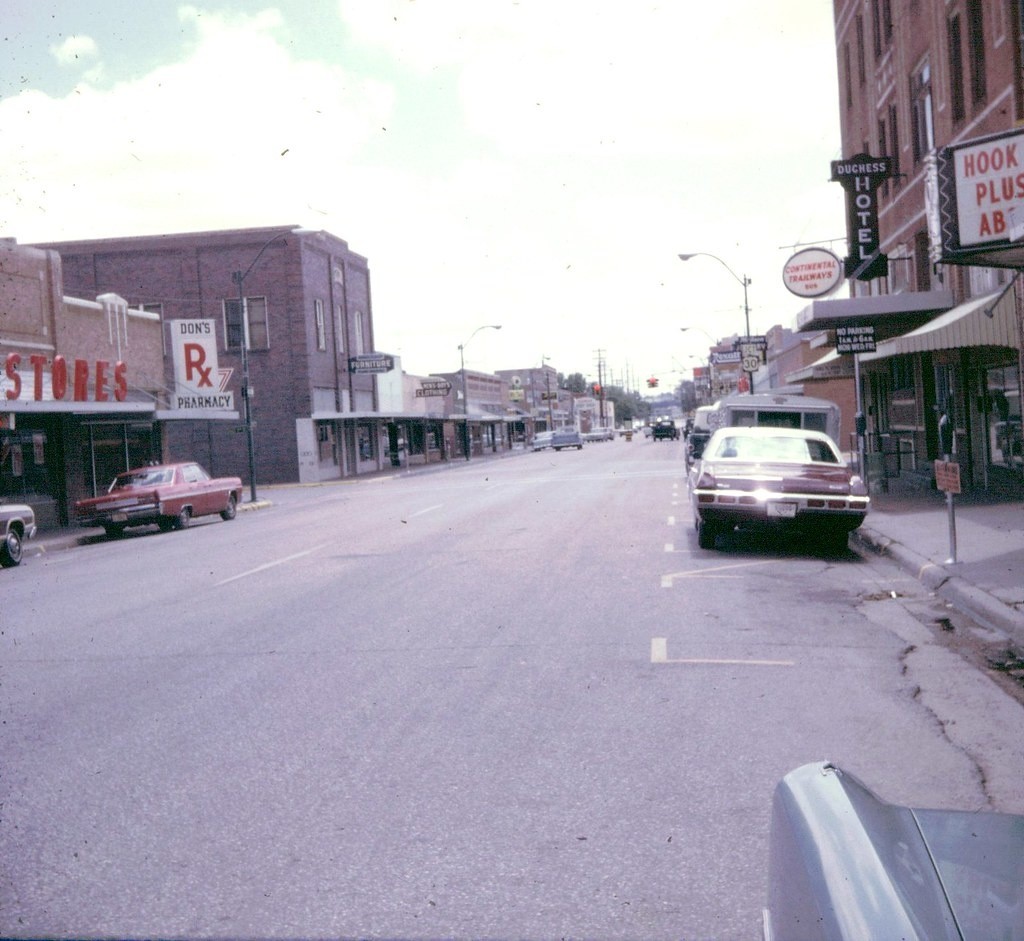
[138,453,159,468]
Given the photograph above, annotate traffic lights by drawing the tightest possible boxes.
[646,374,659,388]
[592,383,604,400]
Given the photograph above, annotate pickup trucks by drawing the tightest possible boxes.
[645,419,680,441]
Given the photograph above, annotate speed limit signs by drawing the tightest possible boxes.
[741,355,759,372]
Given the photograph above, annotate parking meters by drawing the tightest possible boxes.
[939,414,955,563]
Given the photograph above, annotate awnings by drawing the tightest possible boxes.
[784,348,870,383]
[936,236,1024,272]
[857,289,1020,363]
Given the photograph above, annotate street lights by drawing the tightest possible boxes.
[680,323,719,406]
[529,357,551,436]
[232,225,310,501]
[456,322,503,460]
[679,252,754,394]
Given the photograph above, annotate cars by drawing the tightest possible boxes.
[0,504,38,570]
[686,427,871,557]
[532,431,555,451]
[74,461,243,536]
[551,426,584,450]
[587,427,615,443]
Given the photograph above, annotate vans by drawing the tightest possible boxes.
[716,395,841,447]
